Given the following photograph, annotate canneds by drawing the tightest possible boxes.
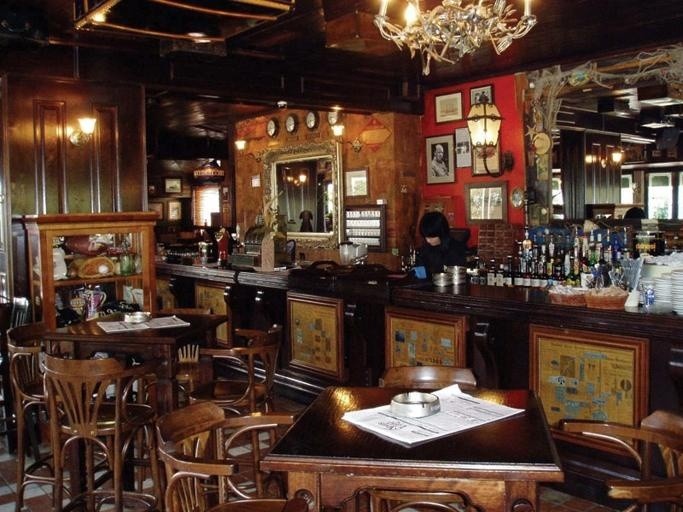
[120,255,134,277]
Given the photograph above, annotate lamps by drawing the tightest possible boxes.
[67,117,95,150]
[374,1,538,77]
[466,102,514,178]
[600,151,622,170]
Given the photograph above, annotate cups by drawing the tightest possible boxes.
[641,279,655,310]
[339,241,353,265]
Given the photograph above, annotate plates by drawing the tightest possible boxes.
[671,269,682,315]
[639,273,671,304]
[52,247,66,280]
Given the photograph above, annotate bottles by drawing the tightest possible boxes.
[485,215,628,290]
[470,257,480,277]
[119,240,132,275]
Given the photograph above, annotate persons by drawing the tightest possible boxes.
[623,207,665,259]
[431,144,450,178]
[413,211,473,275]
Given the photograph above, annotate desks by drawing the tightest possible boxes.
[260,386,565,512]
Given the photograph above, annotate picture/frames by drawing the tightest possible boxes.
[463,180,508,225]
[164,177,183,193]
[469,84,494,108]
[434,91,463,124]
[425,132,456,185]
[343,167,369,199]
[147,202,165,221]
[167,200,182,222]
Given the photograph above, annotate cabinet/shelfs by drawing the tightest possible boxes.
[23,211,160,330]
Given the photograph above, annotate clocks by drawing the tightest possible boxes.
[266,118,279,136]
[284,112,297,134]
[305,111,319,131]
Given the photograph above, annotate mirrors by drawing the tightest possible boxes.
[514,41,683,254]
[261,137,344,250]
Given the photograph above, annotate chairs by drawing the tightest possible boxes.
[6,308,312,512]
[379,367,476,389]
[538,419,682,512]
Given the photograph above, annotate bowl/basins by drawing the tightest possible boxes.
[548,292,586,306]
[433,264,467,288]
[585,293,629,310]
